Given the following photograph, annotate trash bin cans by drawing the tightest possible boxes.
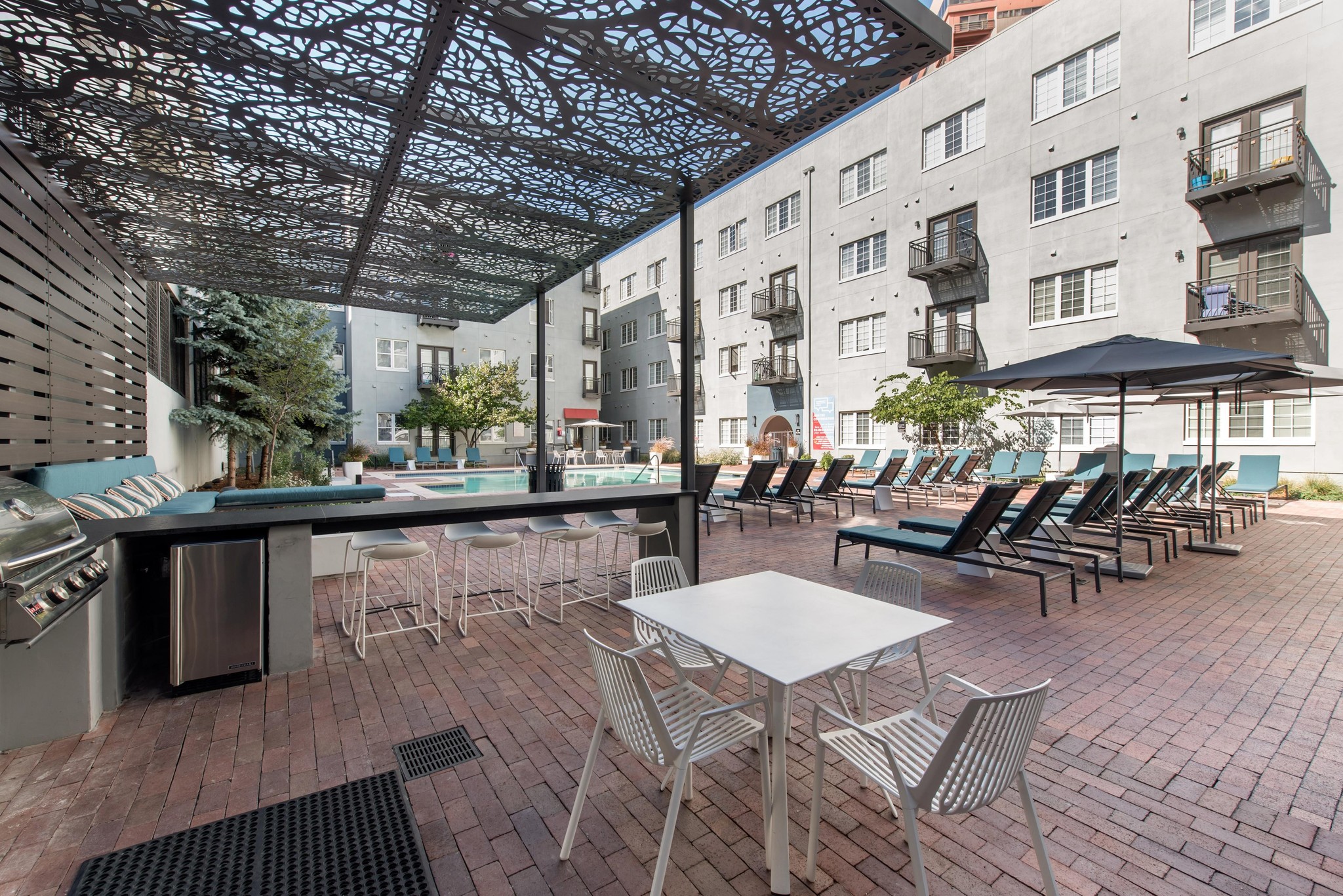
[631,446,641,464]
[526,463,567,520]
[771,446,784,468]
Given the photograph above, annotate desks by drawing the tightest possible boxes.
[612,569,955,895]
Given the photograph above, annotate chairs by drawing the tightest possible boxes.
[808,678,1060,895]
[552,448,628,464]
[630,554,759,707]
[696,441,1282,591]
[387,444,488,470]
[557,628,774,896]
[811,561,948,731]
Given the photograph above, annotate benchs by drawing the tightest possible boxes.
[215,484,387,506]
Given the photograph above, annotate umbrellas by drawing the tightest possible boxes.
[563,419,624,464]
[946,335,1343,579]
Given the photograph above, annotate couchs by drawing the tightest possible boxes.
[34,453,218,523]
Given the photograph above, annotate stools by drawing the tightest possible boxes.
[338,511,676,662]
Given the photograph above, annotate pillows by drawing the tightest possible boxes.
[59,471,184,521]
